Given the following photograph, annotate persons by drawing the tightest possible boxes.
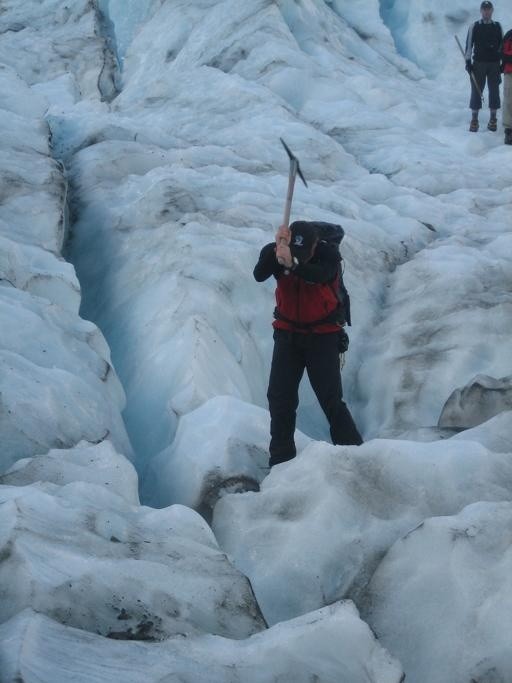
[253,218,363,469]
[463,0,503,133]
[496,28,511,144]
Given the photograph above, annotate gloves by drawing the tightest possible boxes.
[465,62,473,74]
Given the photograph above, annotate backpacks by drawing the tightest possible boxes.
[306,221,353,328]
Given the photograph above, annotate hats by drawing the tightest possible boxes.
[481,1,494,9]
[289,220,319,264]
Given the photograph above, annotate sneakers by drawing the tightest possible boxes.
[469,120,480,133]
[487,119,497,132]
[503,128,512,146]
